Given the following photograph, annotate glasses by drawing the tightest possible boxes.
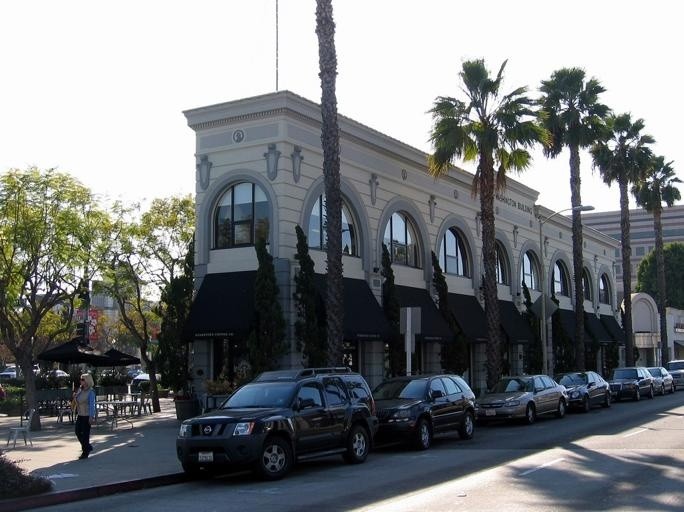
[79,378,85,381]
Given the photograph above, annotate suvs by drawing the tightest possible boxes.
[369,371,478,451]
[176,367,380,482]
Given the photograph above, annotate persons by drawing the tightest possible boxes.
[73,373,95,459]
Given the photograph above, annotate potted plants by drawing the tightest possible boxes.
[207,380,226,393]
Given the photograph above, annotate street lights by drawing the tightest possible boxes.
[535,202,598,376]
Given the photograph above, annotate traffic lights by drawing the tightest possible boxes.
[76,322,86,346]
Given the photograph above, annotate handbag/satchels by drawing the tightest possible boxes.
[71,396,79,413]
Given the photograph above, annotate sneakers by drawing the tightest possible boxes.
[79,444,94,460]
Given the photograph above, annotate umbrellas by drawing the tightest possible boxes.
[90,349,140,366]
[37,336,110,362]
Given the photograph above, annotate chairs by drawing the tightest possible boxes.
[55,391,151,430]
[6,408,36,449]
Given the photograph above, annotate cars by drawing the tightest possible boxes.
[43,370,70,378]
[476,359,683,425]
[79,368,164,392]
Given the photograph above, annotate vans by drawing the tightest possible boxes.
[0,365,40,381]
